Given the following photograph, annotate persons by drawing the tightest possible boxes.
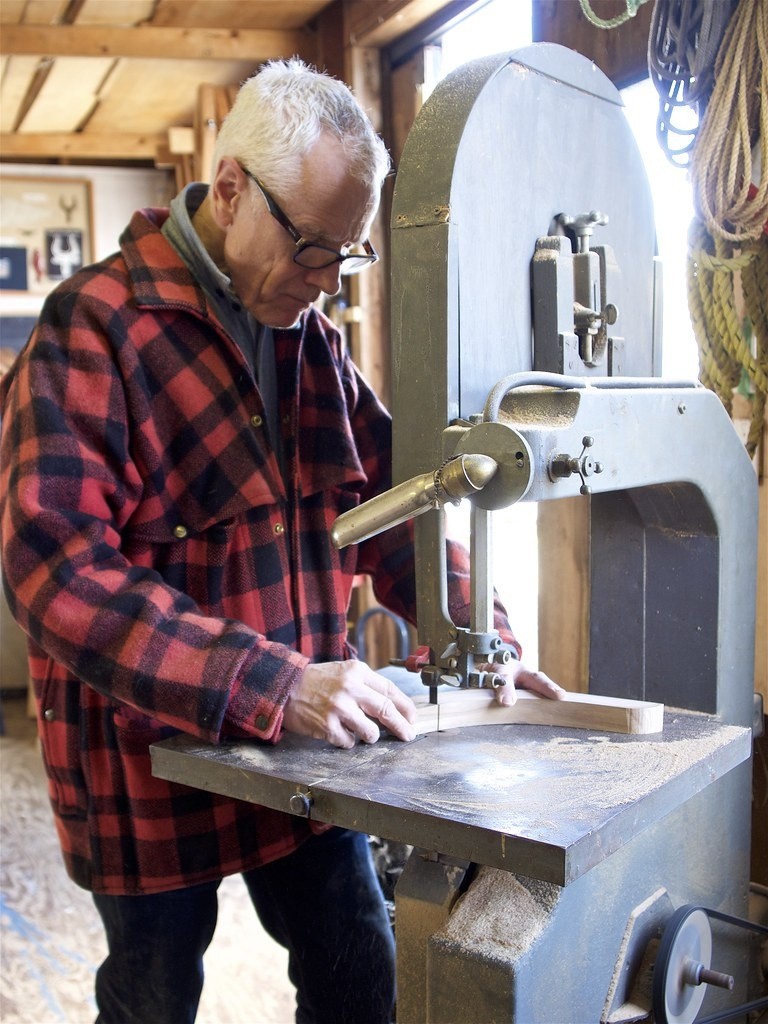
[1,57,570,1024]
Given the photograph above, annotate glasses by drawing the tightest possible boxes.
[243,167,378,277]
